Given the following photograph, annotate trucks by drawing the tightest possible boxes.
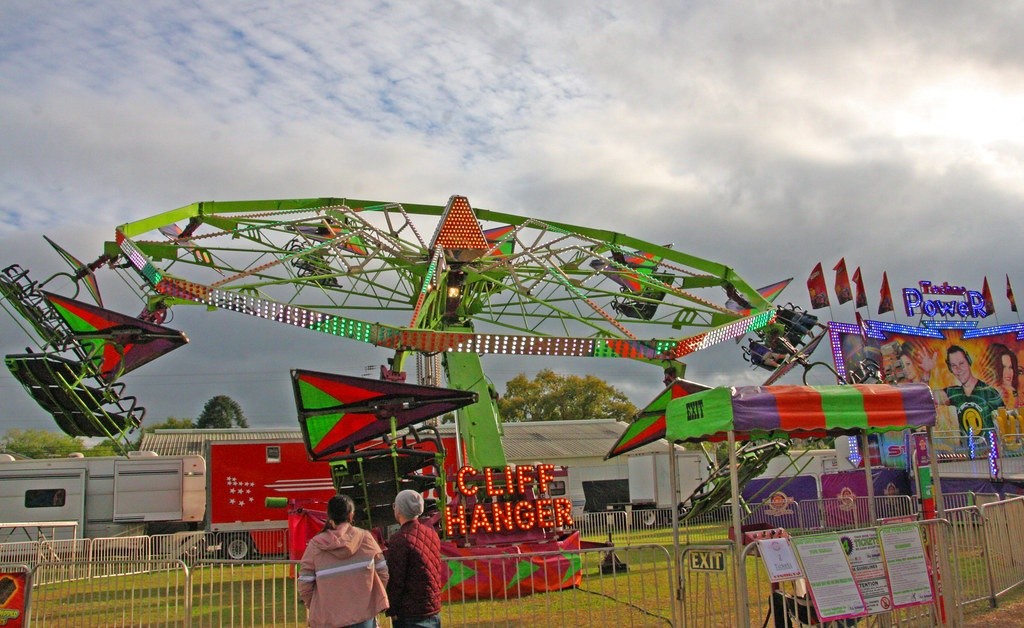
[0,449,207,575]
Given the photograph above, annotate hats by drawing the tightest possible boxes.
[395,489,424,518]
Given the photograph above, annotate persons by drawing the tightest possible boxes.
[384,490,442,628]
[299,494,389,628]
[765,353,790,367]
[900,342,1024,445]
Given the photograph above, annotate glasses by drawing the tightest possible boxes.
[392,503,396,510]
